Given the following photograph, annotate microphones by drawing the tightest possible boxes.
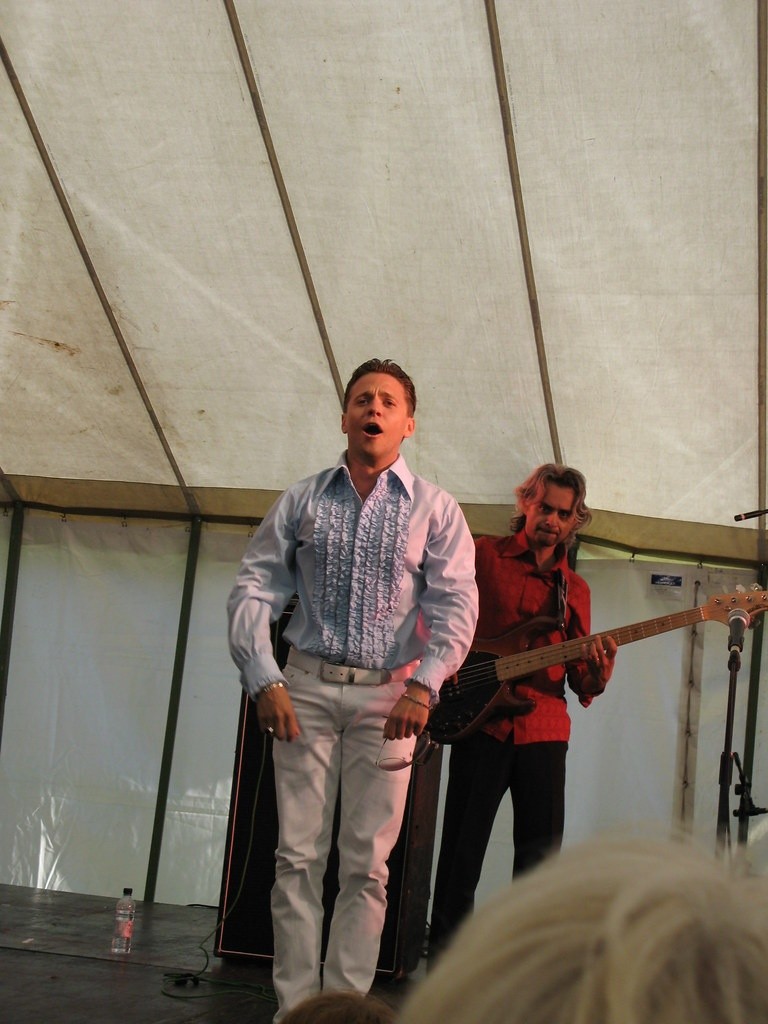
[727,608,750,666]
[734,509,768,521]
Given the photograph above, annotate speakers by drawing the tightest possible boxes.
[213,591,444,983]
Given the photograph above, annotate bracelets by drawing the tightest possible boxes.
[255,681,286,699]
[402,693,431,708]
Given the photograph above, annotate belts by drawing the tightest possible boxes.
[284,645,423,686]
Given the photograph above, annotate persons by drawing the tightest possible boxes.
[281,830,768,1024]
[425,463,618,976]
[226,358,478,1024]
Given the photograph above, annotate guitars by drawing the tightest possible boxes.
[426,583,768,745]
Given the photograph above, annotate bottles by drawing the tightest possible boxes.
[112,888,136,954]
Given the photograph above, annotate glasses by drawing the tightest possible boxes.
[376,715,431,771]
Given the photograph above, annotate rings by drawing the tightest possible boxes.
[593,658,599,664]
[264,720,275,736]
